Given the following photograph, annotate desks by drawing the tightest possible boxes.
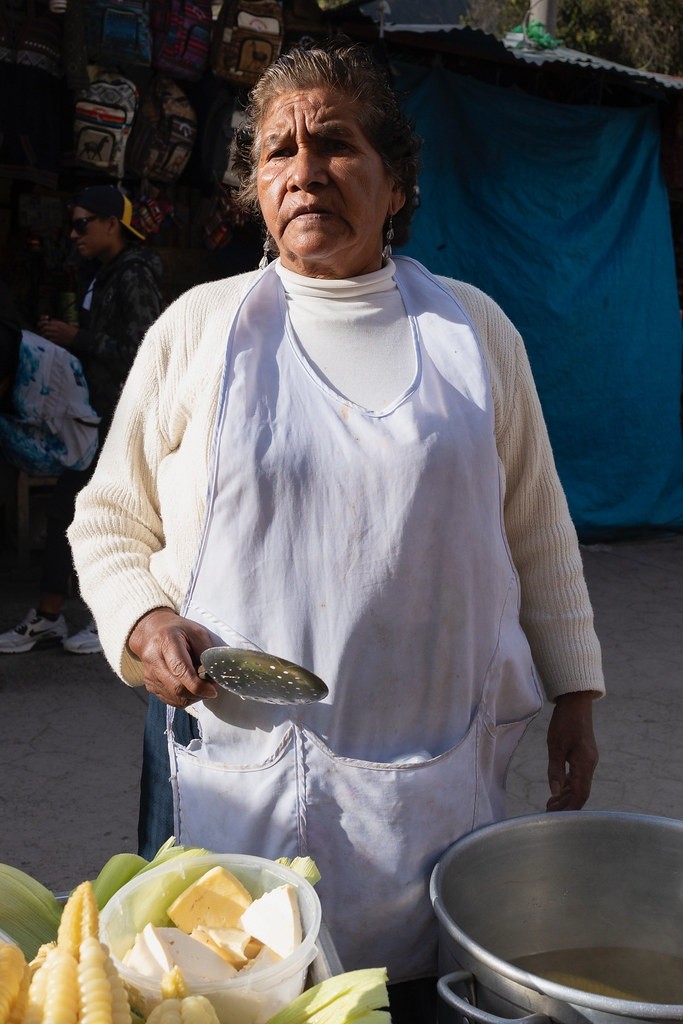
[386,976,442,1024]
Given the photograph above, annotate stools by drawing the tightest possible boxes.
[13,469,59,575]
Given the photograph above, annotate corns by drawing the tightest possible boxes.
[1,881,220,1024]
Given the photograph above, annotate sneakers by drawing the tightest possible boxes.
[1,609,68,654]
[65,619,104,658]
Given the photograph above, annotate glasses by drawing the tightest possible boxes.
[69,214,99,232]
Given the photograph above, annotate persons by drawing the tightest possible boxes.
[36,183,187,442]
[64,43,610,991]
[0,326,111,656]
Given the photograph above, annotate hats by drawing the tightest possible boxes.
[77,184,147,240]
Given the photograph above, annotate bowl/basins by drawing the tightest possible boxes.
[95,851,323,1023]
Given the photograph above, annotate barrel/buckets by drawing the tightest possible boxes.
[427,798,682,1024]
